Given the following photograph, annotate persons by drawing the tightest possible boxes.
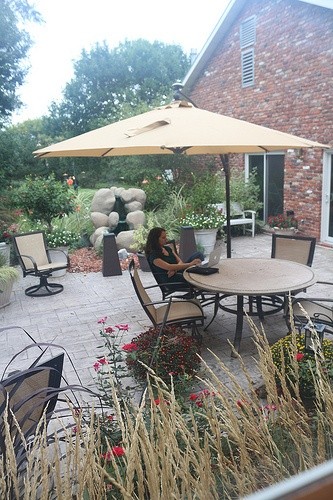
[145,227,204,289]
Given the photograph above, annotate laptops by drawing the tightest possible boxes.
[193,248,222,268]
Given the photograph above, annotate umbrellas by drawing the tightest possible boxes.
[34,83,330,258]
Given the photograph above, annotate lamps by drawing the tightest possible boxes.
[305,314,327,356]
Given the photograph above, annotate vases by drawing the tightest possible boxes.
[274,227,296,235]
[194,228,220,254]
[47,247,70,277]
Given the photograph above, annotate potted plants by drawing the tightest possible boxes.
[0,265,18,309]
[131,225,153,271]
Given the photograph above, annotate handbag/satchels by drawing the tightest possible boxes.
[186,266,219,275]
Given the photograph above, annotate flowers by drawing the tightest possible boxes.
[180,207,227,228]
[46,229,82,248]
[73,313,332,481]
[269,214,298,228]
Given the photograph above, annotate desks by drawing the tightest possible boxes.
[184,257,318,357]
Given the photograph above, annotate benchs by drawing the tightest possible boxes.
[208,202,257,238]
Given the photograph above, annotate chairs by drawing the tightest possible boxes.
[0,351,65,469]
[14,232,70,297]
[128,233,333,361]
